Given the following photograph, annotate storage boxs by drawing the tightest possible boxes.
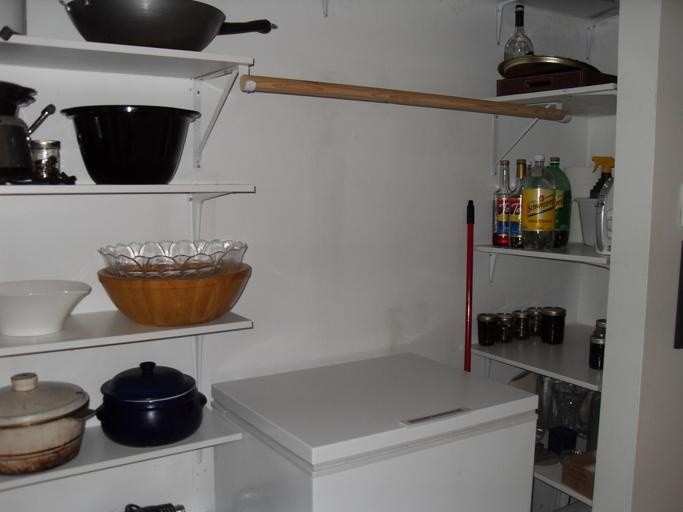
[560,450,596,499]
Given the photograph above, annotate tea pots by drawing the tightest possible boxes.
[0,81,55,185]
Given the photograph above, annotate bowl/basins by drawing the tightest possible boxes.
[0,281,92,341]
[97,264,252,326]
[60,105,201,184]
[97,239,248,277]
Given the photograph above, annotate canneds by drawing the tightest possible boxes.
[588,335,605,371]
[495,312,513,345]
[27,138,62,185]
[527,306,543,337]
[592,326,608,339]
[539,305,567,345]
[477,312,495,347]
[511,308,528,341]
[594,318,606,328]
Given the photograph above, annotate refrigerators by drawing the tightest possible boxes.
[210,355,539,510]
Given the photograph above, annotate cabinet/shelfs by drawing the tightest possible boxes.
[0,30,255,512]
[470,80,618,511]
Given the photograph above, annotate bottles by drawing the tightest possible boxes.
[478,304,603,368]
[504,6,534,59]
[493,156,571,252]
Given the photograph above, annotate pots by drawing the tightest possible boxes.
[96,362,207,448]
[0,373,96,475]
[57,1,277,52]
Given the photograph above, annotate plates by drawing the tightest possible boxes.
[498,56,599,79]
